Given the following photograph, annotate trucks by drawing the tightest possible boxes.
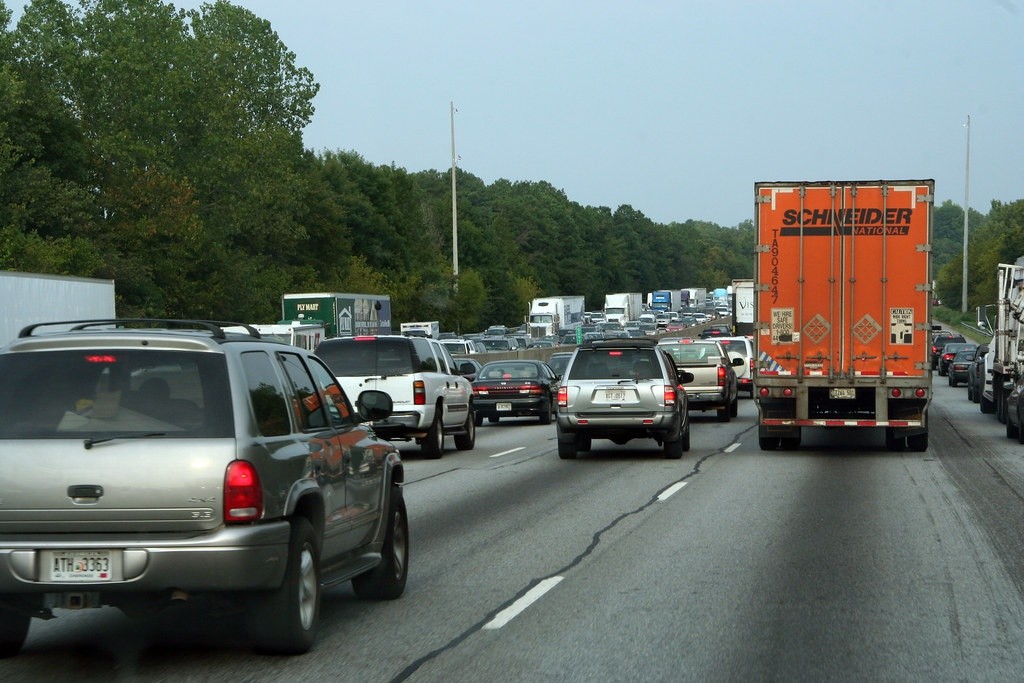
[750,176,944,455]
[732,278,756,337]
[1,271,116,361]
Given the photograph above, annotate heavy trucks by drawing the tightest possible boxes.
[978,252,1024,445]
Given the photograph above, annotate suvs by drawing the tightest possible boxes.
[699,335,754,400]
[551,336,695,461]
[311,333,478,459]
[0,316,409,653]
[656,337,745,422]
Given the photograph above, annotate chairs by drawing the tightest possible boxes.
[632,360,652,377]
[488,371,502,378]
[587,362,610,379]
[519,370,532,377]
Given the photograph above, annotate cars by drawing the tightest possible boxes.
[468,360,567,426]
[930,325,969,371]
[548,351,573,381]
[966,343,990,404]
[208,285,736,358]
[947,349,978,388]
[937,342,980,376]
[448,355,483,382]
